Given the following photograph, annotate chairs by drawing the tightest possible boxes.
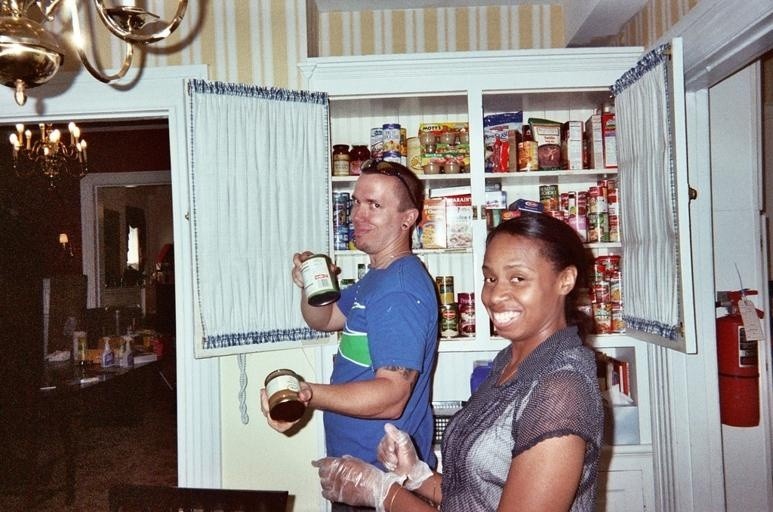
[106,487,299,512]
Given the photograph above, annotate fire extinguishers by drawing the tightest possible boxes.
[714,289,765,428]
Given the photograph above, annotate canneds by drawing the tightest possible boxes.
[349,144,371,176]
[332,144,349,176]
[457,292,474,336]
[261,368,306,413]
[540,178,622,242]
[439,304,459,338]
[436,275,454,304]
[300,254,341,308]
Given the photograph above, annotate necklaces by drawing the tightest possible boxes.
[495,361,519,389]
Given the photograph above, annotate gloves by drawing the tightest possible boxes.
[312,454,402,512]
[377,422,434,491]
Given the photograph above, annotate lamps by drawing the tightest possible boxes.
[4,121,92,188]
[0,0,190,108]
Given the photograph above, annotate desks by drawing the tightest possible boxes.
[3,355,163,504]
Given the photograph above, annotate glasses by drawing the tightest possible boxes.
[361,159,417,208]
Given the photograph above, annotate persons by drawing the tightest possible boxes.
[311,213,605,512]
[258,160,439,511]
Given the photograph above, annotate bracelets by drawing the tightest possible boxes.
[390,487,404,511]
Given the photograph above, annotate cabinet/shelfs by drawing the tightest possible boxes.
[167,37,698,512]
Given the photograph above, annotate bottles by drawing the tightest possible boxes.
[77,336,87,366]
[407,137,424,175]
[518,124,539,171]
[370,128,383,165]
[383,123,401,164]
[401,128,407,168]
